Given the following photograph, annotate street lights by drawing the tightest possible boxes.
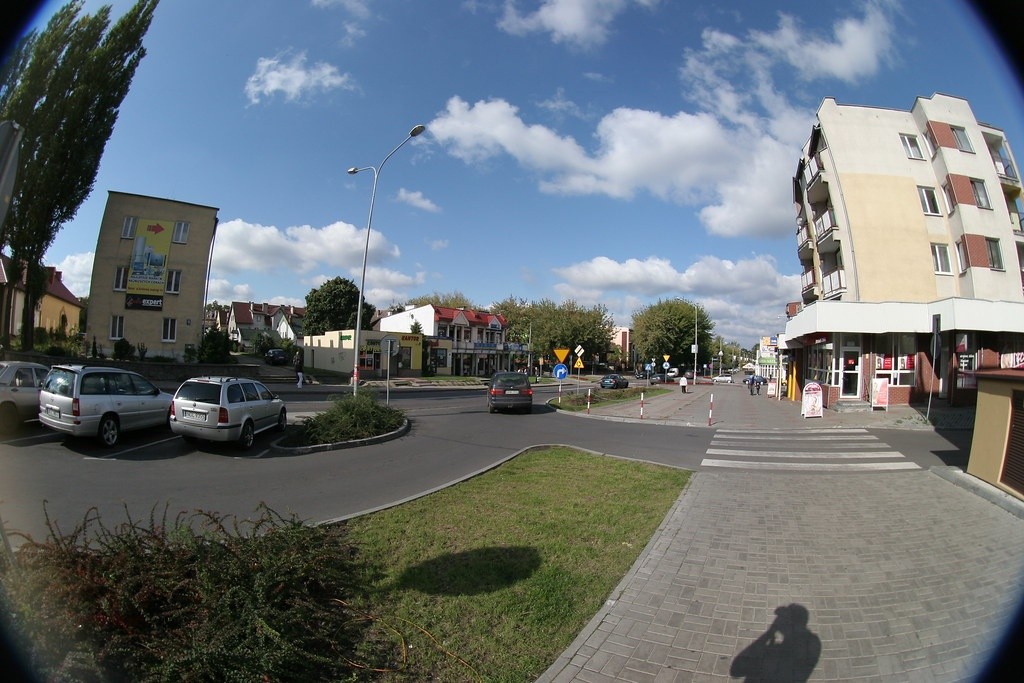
[675,296,697,385]
[347,124,426,400]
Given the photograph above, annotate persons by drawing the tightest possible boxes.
[755,383,760,395]
[293,351,304,389]
[679,375,687,393]
[750,376,755,395]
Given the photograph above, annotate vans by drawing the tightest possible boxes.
[668,368,680,376]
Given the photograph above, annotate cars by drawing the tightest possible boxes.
[0,360,57,436]
[484,372,533,413]
[266,348,288,365]
[170,375,290,449]
[685,370,694,379]
[782,379,788,386]
[636,369,655,380]
[35,363,178,448]
[649,373,674,385]
[743,376,767,386]
[600,373,629,389]
[712,374,734,384]
